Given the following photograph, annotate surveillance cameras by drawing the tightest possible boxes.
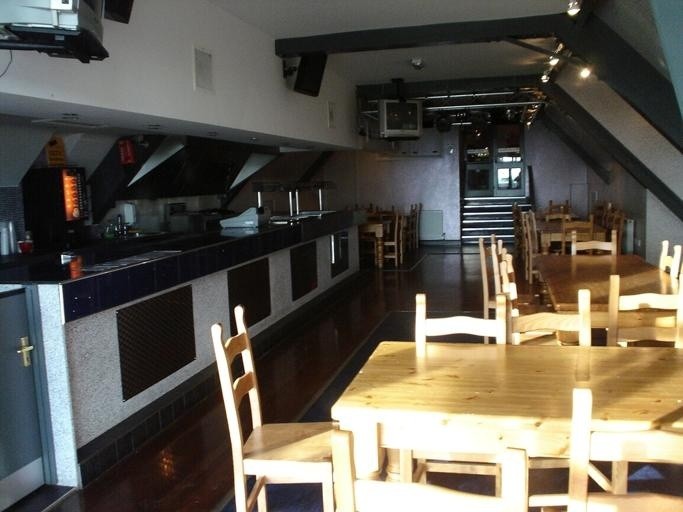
[412,59,426,70]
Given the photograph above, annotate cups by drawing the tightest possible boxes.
[1,222,33,254]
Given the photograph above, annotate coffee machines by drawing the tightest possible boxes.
[24,167,95,280]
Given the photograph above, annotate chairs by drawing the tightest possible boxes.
[562,384,682,511]
[413,290,509,346]
[338,198,427,272]
[505,198,632,284]
[328,428,529,512]
[477,233,682,348]
[211,302,338,510]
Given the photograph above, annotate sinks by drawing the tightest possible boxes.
[292,210,336,219]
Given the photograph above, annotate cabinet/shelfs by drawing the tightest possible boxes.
[0,293,46,512]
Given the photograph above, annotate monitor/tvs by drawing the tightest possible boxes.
[378,98,423,141]
[0,0,107,46]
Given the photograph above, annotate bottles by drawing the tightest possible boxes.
[69,257,80,278]
[115,214,128,237]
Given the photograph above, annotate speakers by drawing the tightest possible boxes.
[294,46,327,99]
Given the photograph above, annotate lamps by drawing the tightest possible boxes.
[526,0,582,126]
[411,58,423,70]
